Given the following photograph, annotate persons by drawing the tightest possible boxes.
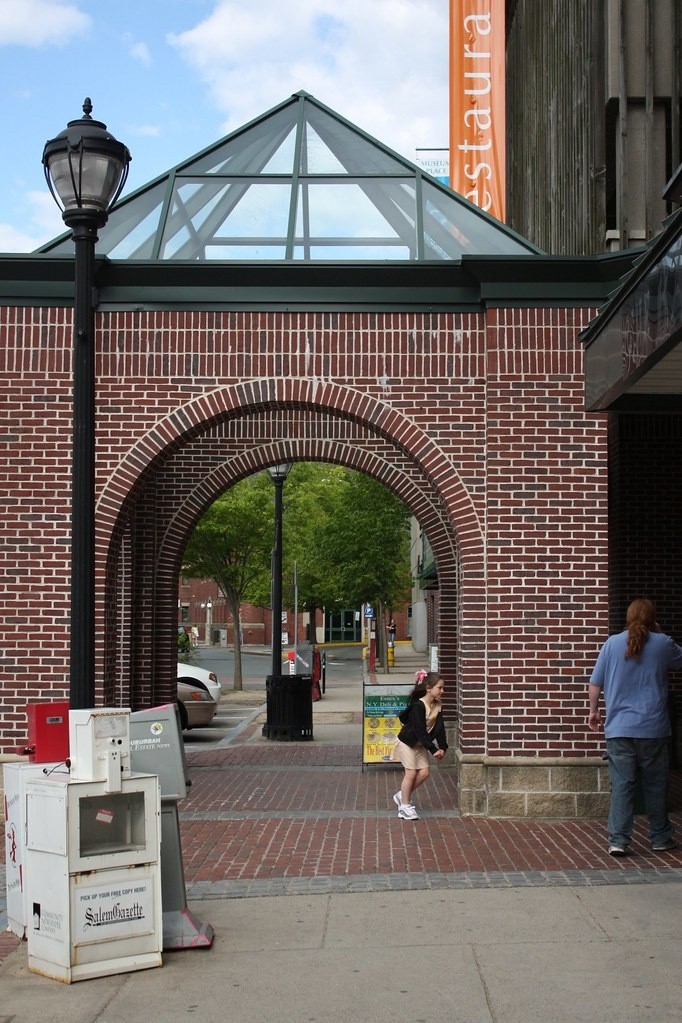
[191,624,199,647]
[178,623,190,653]
[392,672,448,820]
[386,619,397,647]
[588,600,682,855]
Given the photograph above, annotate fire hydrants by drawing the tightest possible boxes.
[387,641,396,669]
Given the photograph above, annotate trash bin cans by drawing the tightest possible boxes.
[265,675,314,742]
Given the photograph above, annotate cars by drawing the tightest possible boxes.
[176,661,222,733]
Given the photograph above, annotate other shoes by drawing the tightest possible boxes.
[653,839,675,850]
[608,844,625,855]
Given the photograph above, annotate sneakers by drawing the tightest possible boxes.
[397,804,420,819]
[393,790,413,806]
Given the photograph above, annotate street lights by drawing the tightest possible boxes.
[41,95,136,711]
[201,597,213,645]
[267,459,294,675]
